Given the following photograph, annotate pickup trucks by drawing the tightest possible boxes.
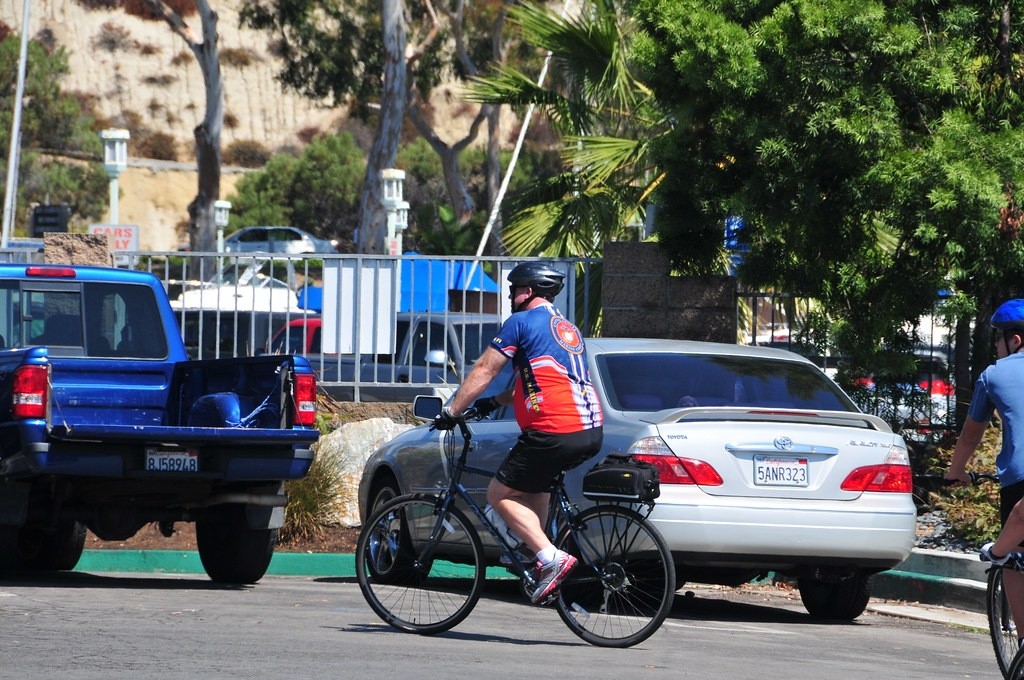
[0,261,320,587]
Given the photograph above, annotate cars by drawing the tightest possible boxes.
[156,251,517,424]
[743,327,955,452]
[356,335,919,621]
[212,225,339,256]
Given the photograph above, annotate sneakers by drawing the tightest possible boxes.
[500,544,536,564]
[531,548,578,604]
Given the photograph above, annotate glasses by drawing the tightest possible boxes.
[510,284,531,295]
[995,332,1020,342]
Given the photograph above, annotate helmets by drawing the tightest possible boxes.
[507,263,566,298]
[990,298,1024,329]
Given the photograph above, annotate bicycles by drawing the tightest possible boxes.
[941,469,1024,680]
[977,551,1024,680]
[352,406,677,648]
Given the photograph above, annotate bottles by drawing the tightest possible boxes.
[483,505,525,551]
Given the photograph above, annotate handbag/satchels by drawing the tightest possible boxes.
[582,451,660,503]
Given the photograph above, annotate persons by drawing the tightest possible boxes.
[943,299,1024,642]
[434,263,605,603]
[980,498,1024,646]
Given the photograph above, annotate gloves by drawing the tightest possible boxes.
[435,409,463,431]
[979,542,1012,566]
[472,395,502,422]
